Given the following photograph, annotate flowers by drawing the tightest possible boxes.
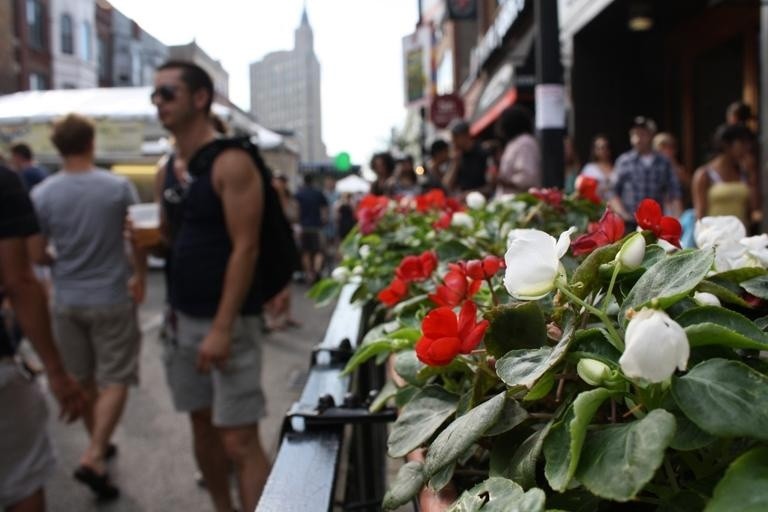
[304,175,767,510]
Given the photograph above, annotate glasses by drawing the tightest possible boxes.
[150,87,176,105]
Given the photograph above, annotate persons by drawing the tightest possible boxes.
[151,59,275,511]
[369,106,542,197]
[262,173,356,334]
[564,101,763,229]
[0,114,147,511]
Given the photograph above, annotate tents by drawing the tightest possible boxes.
[337,173,370,194]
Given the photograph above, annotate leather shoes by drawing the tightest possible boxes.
[74,465,117,501]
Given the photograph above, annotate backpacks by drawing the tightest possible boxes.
[210,136,303,316]
